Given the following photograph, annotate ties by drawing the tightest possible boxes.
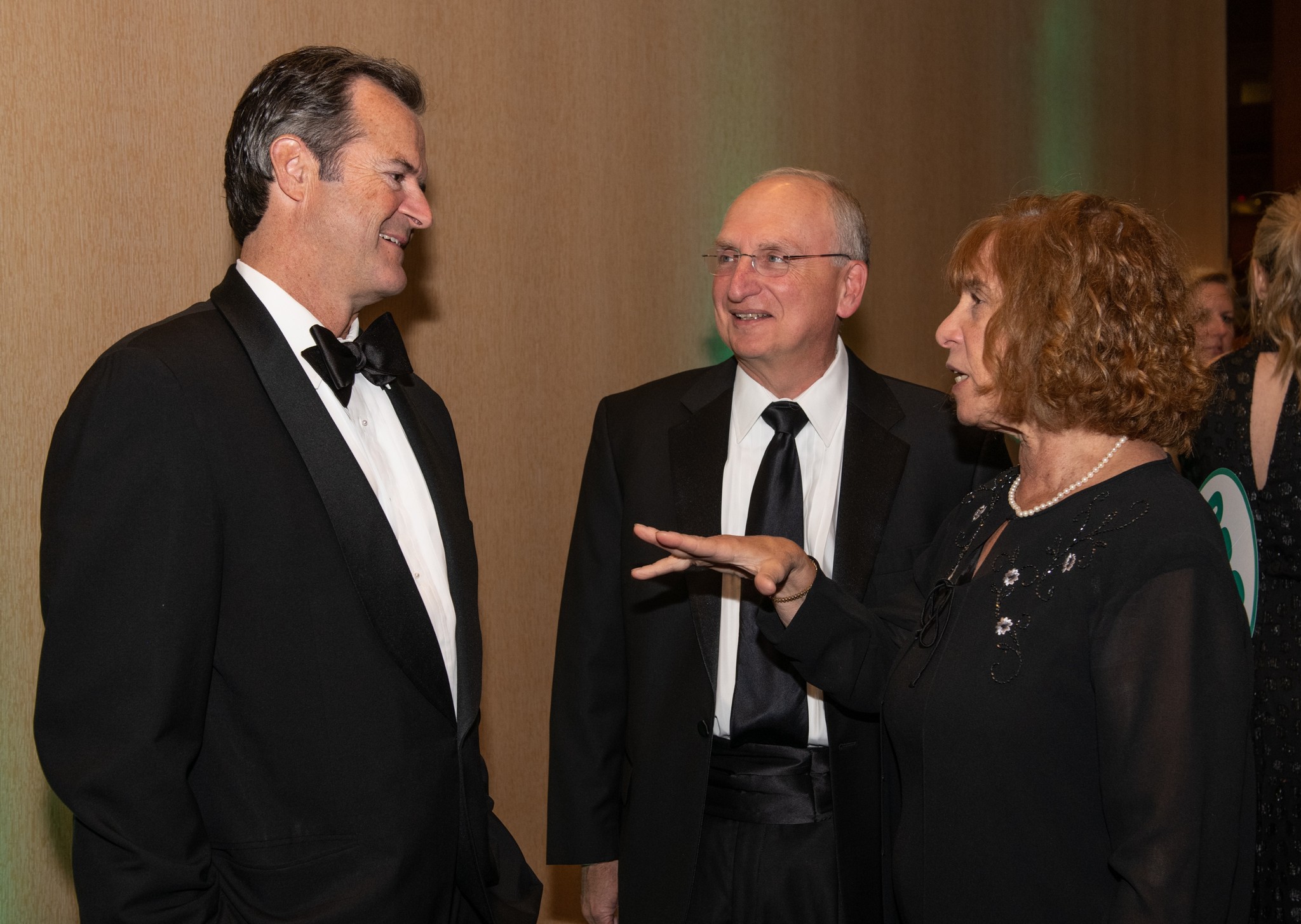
[729,401,809,747]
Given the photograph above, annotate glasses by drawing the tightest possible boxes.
[701,245,852,277]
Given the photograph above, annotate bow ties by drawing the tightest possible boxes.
[300,312,414,407]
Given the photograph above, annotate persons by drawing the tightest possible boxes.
[629,193,1259,924]
[1182,263,1235,368]
[31,43,546,923]
[1175,195,1301,923]
[547,166,1017,924]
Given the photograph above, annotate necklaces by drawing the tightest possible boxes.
[1007,432,1130,517]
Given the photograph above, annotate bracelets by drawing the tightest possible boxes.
[768,557,817,603]
